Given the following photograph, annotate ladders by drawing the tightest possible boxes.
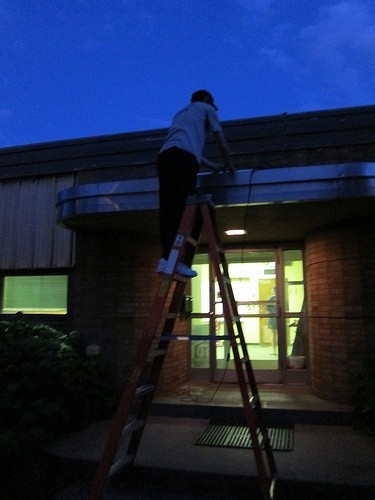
[84,185,281,500]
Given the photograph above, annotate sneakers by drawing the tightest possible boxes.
[156,258,198,277]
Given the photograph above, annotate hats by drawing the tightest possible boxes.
[191,89,219,111]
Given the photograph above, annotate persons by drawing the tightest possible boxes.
[266,288,289,354]
[155,89,235,278]
